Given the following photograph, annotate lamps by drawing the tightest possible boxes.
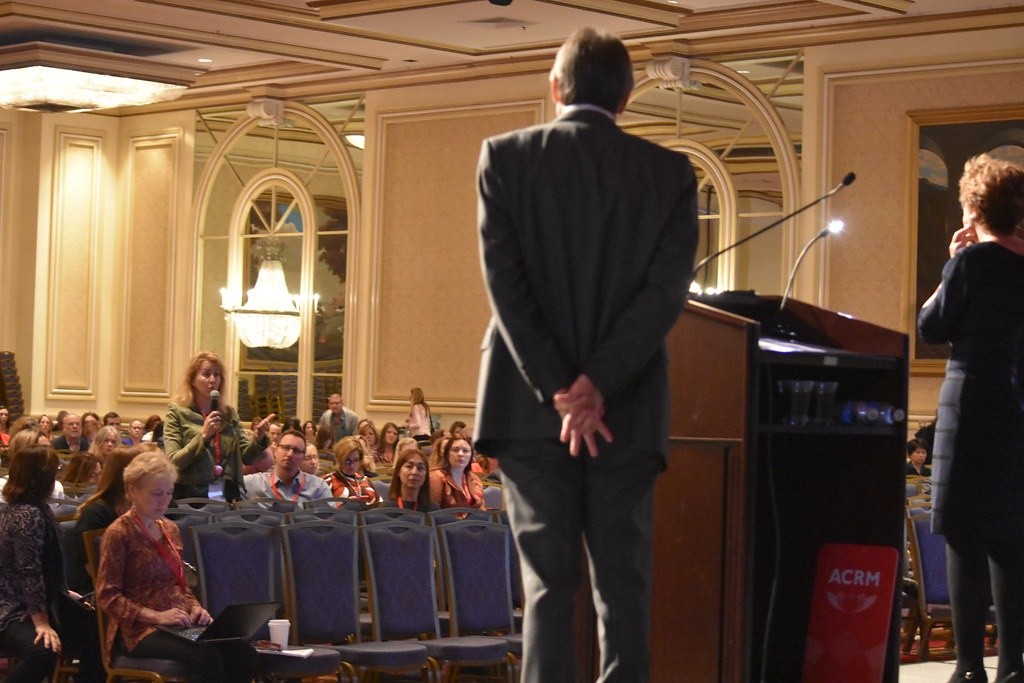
[0,40,212,115]
[217,231,321,349]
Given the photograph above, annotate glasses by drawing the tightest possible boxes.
[343,459,361,465]
[277,444,306,454]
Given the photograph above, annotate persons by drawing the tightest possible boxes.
[915,154,1024,683]
[319,394,359,444]
[916,422,935,463]
[0,405,490,599]
[405,388,431,441]
[473,26,700,683]
[0,445,106,683]
[164,351,275,508]
[96,452,258,683]
[906,438,930,476]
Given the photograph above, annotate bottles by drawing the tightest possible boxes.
[843,402,904,426]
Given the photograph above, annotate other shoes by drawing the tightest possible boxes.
[957,669,987,683]
[998,670,1024,683]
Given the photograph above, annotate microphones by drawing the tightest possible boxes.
[210,390,220,416]
[693,171,857,278]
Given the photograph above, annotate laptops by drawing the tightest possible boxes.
[153,601,283,646]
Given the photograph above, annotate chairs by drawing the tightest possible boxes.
[48,468,998,683]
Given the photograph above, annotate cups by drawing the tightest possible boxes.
[763,379,838,427]
[268,619,291,650]
